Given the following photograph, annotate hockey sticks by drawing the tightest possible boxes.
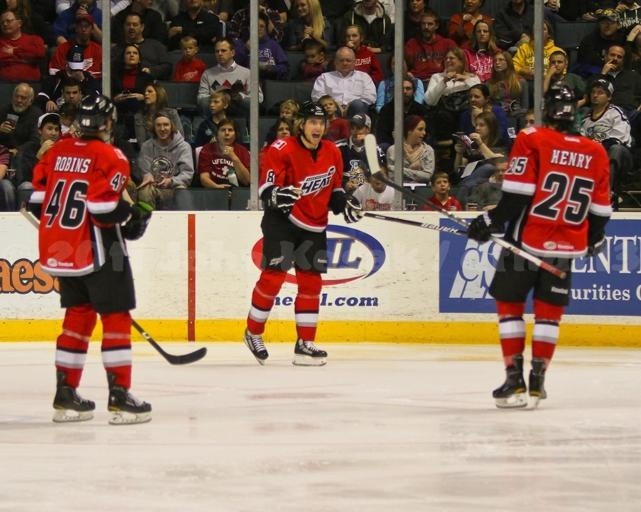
[364,133,567,280]
[19,206,207,365]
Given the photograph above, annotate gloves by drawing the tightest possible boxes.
[467,209,505,241]
[266,186,302,214]
[120,201,152,240]
[338,192,362,223]
[585,234,608,257]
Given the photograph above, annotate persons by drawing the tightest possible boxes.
[26,93,156,416]
[0,0,640,214]
[465,82,616,401]
[244,98,367,361]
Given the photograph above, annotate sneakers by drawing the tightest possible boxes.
[53,386,94,411]
[245,328,267,359]
[108,385,151,412]
[295,338,327,357]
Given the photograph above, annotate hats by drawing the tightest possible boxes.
[591,78,615,97]
[350,113,372,129]
[38,112,61,128]
[152,112,170,120]
[597,8,620,22]
[76,14,93,25]
[67,45,85,70]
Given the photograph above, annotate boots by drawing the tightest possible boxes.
[529,370,547,398]
[492,365,526,397]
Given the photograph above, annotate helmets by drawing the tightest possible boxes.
[79,95,114,128]
[303,103,326,117]
[543,84,576,121]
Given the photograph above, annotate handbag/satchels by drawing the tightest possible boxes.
[166,103,198,113]
